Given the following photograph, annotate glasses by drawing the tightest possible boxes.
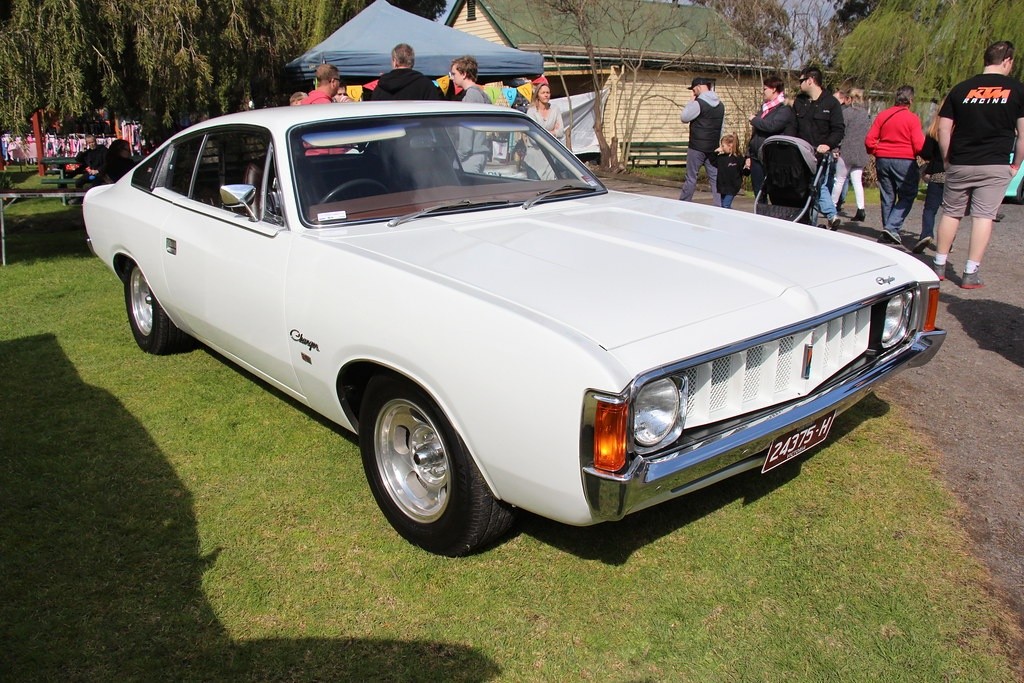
[338,93,347,96]
[329,78,340,83]
[799,78,809,84]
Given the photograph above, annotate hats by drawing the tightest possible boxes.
[687,77,707,91]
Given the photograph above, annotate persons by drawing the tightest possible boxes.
[301,64,345,156]
[358,44,444,102]
[709,132,752,209]
[678,76,726,207]
[933,40,1024,289]
[830,88,873,222]
[448,55,494,175]
[105,140,140,183]
[832,88,850,215]
[290,91,308,106]
[68,133,109,205]
[792,66,846,232]
[864,85,957,254]
[331,86,354,102]
[523,83,564,180]
[747,76,794,205]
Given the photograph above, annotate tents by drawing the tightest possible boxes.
[284,0,543,79]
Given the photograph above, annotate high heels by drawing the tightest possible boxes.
[851,208,865,222]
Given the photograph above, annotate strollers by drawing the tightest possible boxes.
[752,134,833,228]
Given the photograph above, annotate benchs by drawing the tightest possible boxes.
[0,189,94,265]
[620,140,690,171]
[40,170,77,184]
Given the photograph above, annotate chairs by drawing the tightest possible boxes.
[247,138,460,217]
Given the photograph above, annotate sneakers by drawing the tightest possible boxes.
[962,269,985,289]
[933,260,946,280]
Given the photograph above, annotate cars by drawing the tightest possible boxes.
[80,96,949,560]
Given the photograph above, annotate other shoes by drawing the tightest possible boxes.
[881,229,902,244]
[831,219,841,231]
[911,236,932,254]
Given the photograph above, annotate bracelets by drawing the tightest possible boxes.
[1009,164,1020,170]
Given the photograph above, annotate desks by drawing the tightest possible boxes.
[40,157,78,190]
